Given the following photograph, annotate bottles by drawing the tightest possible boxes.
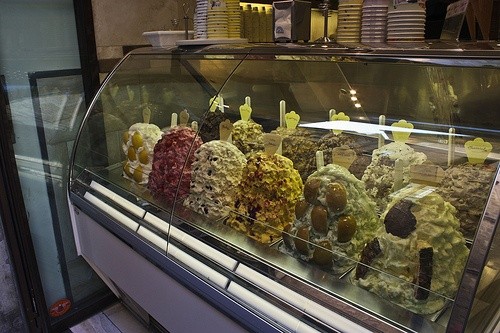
[240,3,273,44]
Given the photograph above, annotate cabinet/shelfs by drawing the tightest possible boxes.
[66,44,500,333]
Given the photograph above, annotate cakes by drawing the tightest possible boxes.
[119,110,497,313]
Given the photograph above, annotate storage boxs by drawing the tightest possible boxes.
[336,0,426,48]
[192,0,272,42]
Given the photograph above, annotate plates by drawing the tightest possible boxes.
[334,2,426,43]
[174,37,248,47]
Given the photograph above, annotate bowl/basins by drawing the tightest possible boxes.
[192,1,240,39]
[140,30,192,48]
